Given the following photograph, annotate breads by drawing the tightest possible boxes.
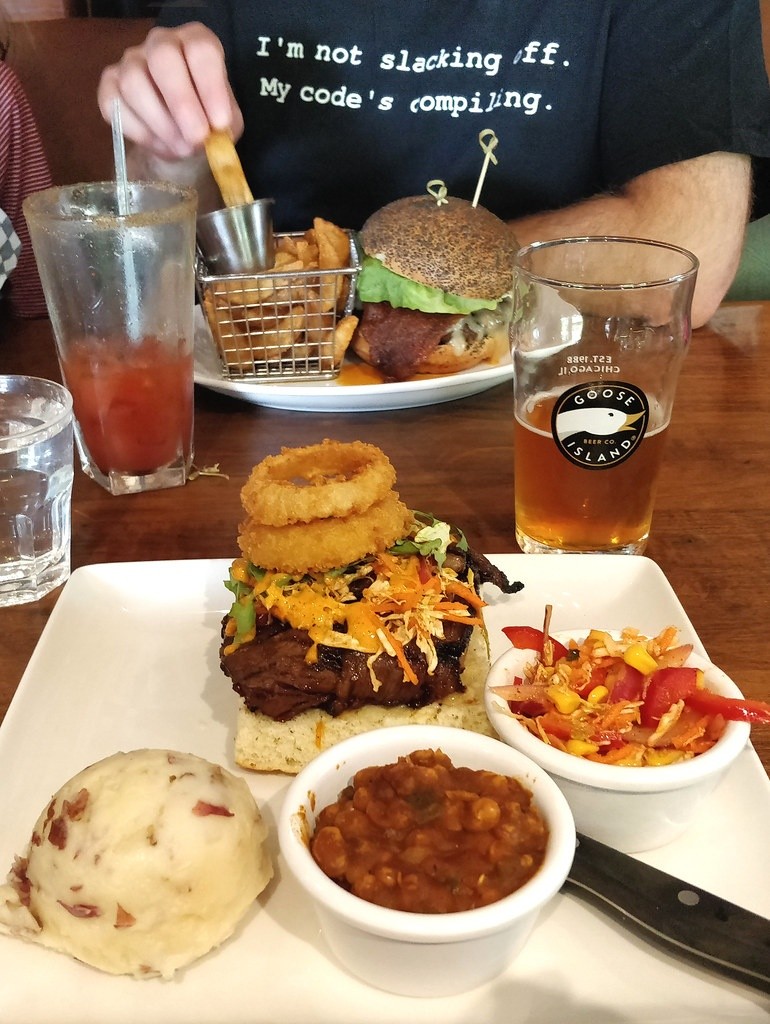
[231,623,498,776]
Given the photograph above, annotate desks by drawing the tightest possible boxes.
[0,298,770,785]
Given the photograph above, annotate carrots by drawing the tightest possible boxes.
[225,550,487,687]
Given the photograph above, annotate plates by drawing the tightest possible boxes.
[194,282,584,412]
[0,554,770,1024]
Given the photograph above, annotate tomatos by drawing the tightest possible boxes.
[493,624,770,729]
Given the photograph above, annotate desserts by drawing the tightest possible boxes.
[0,749,275,979]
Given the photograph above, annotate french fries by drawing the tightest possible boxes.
[203,130,254,205]
[203,216,360,373]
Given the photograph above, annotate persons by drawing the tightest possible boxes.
[97,0,770,329]
[0,55,55,320]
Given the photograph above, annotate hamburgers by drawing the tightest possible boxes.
[350,194,526,384]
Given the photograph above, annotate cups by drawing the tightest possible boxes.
[0,375,72,608]
[279,724,574,998]
[22,179,198,492]
[483,632,753,853]
[511,235,700,555]
[196,192,274,274]
